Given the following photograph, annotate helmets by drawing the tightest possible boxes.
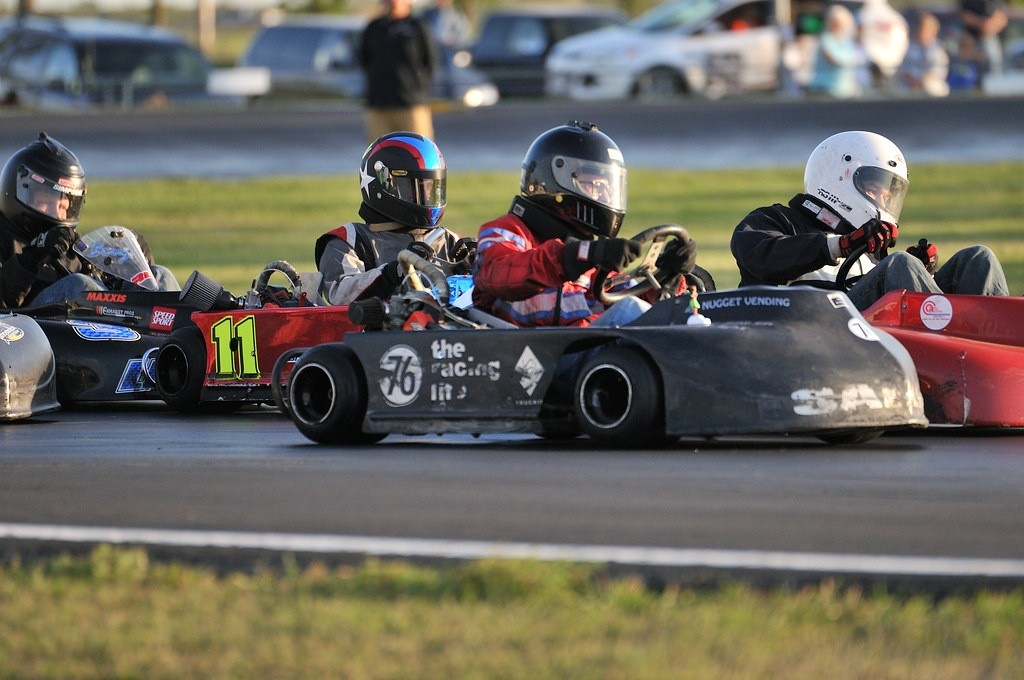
[358,131,448,228]
[804,130,909,234]
[0,132,87,238]
[508,119,627,243]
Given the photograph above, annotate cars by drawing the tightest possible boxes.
[240,13,502,109]
[402,5,629,100]
[0,16,271,113]
[541,0,912,104]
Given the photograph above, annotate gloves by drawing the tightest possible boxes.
[382,241,435,286]
[135,232,155,266]
[655,238,697,276]
[22,225,81,276]
[571,238,641,272]
[839,218,899,260]
[906,238,939,275]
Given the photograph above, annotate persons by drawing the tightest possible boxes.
[0,130,182,314]
[729,131,1010,313]
[357,0,474,143]
[776,0,1024,96]
[478,120,687,329]
[314,130,475,307]
